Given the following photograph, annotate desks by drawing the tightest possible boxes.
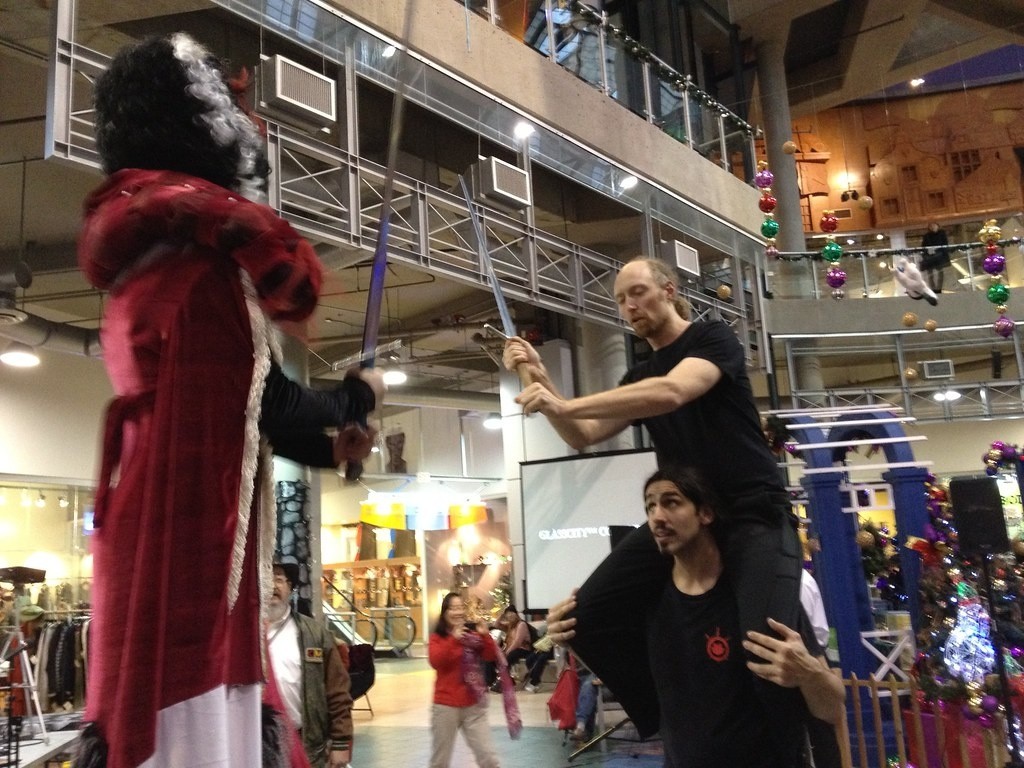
[0,730,86,768]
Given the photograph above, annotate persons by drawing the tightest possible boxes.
[543,466,846,768]
[921,220,952,293]
[267,563,354,768]
[426,592,496,768]
[74,28,388,768]
[545,649,602,741]
[490,604,556,693]
[499,253,831,673]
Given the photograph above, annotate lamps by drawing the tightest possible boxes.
[380,350,408,385]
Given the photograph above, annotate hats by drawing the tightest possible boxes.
[504,604,518,614]
[272,553,299,590]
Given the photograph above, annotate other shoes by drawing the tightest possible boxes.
[525,679,541,693]
[570,728,588,742]
[490,677,504,694]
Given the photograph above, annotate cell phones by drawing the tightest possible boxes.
[464,622,477,630]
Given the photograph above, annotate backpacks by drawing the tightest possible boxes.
[515,620,540,649]
